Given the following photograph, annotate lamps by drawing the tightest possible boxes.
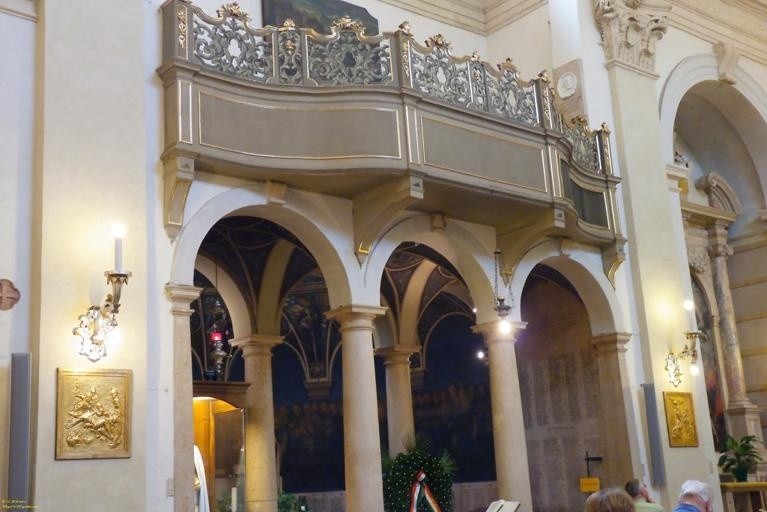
[211,299,230,378]
[664,299,703,388]
[70,222,133,364]
[490,240,517,338]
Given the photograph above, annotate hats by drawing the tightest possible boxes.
[680,480,710,503]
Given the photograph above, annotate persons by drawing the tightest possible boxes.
[64,383,123,447]
[669,397,694,440]
[671,480,713,512]
[625,479,665,512]
[584,488,636,512]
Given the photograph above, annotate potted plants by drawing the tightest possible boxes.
[718,433,766,483]
